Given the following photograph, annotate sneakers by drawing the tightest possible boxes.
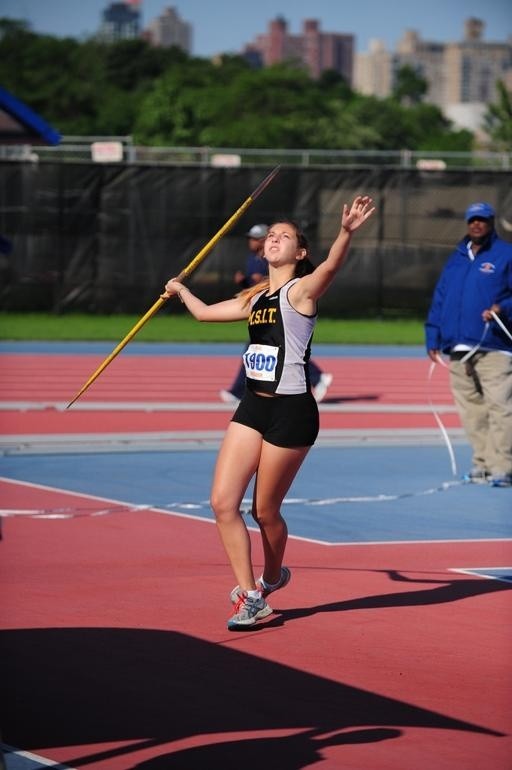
[467,469,512,487]
[313,373,334,403]
[220,389,240,403]
[226,565,291,629]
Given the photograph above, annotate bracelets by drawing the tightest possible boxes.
[177,288,190,303]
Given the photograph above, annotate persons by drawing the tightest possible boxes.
[424,203,512,486]
[220,224,333,403]
[164,197,375,628]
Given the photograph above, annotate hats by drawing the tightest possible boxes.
[464,202,495,224]
[245,224,269,239]
